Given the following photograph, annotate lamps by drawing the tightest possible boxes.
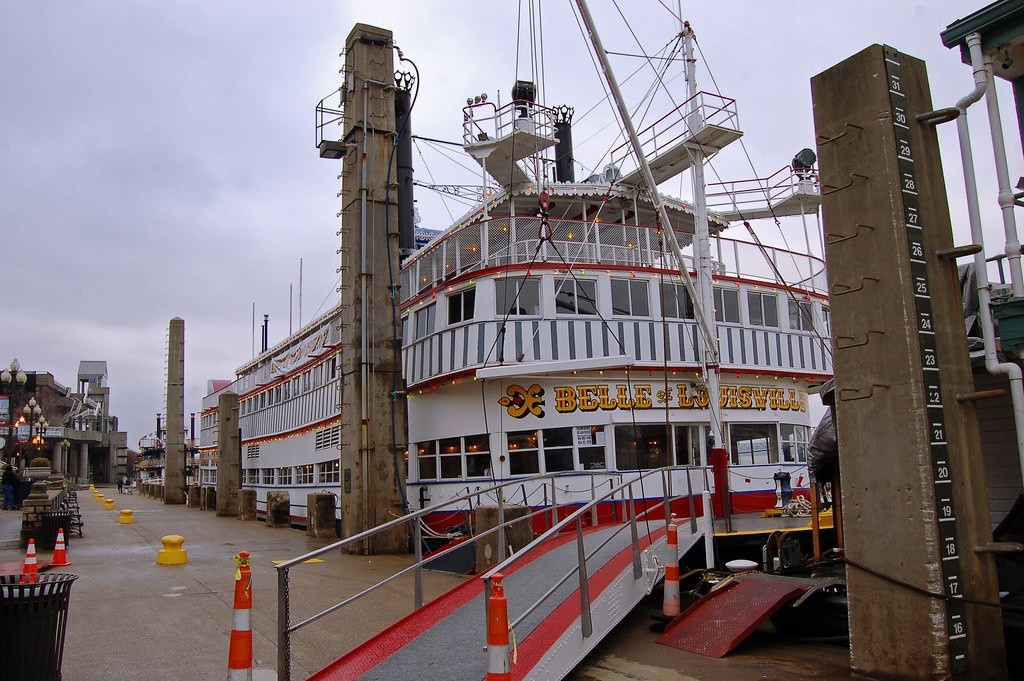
[319,141,348,160]
[478,133,489,141]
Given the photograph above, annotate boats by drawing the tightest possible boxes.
[131,411,199,486]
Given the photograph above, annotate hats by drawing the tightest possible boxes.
[4,466,12,469]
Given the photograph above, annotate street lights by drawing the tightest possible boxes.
[35,415,49,457]
[0,358,27,465]
[22,396,42,468]
[60,439,71,475]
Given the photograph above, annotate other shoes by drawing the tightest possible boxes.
[2,508,8,510]
[11,505,19,510]
[119,492,120,493]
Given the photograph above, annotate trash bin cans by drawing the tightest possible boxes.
[1,574,80,681]
[39,510,72,551]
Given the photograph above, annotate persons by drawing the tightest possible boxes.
[116,473,124,497]
[2,466,19,510]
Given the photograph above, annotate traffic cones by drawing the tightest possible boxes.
[48,527,72,566]
[19,539,44,585]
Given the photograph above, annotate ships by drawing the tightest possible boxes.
[198,0,835,578]
